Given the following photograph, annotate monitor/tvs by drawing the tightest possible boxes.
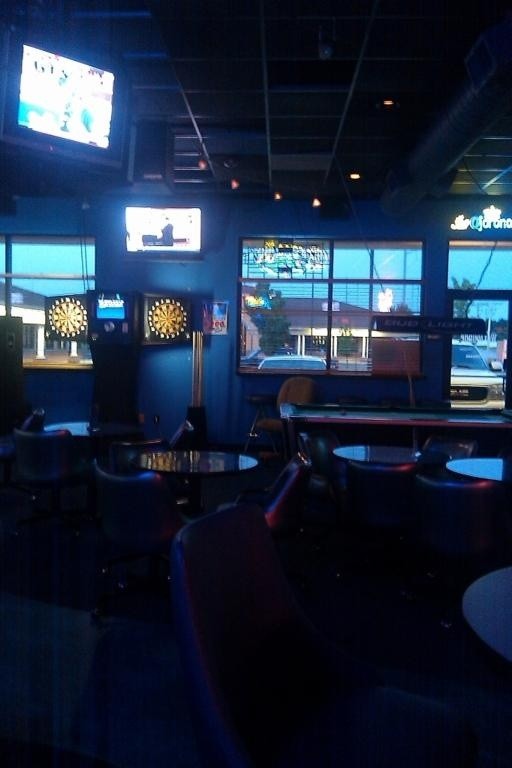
[122,204,206,261]
[0,30,132,172]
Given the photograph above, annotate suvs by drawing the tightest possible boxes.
[449,337,506,416]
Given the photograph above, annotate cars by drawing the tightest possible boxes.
[253,352,328,371]
[240,344,296,366]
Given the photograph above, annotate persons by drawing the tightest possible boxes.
[161,215,175,248]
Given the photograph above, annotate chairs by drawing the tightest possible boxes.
[169,499,480,765]
[2,374,509,662]
